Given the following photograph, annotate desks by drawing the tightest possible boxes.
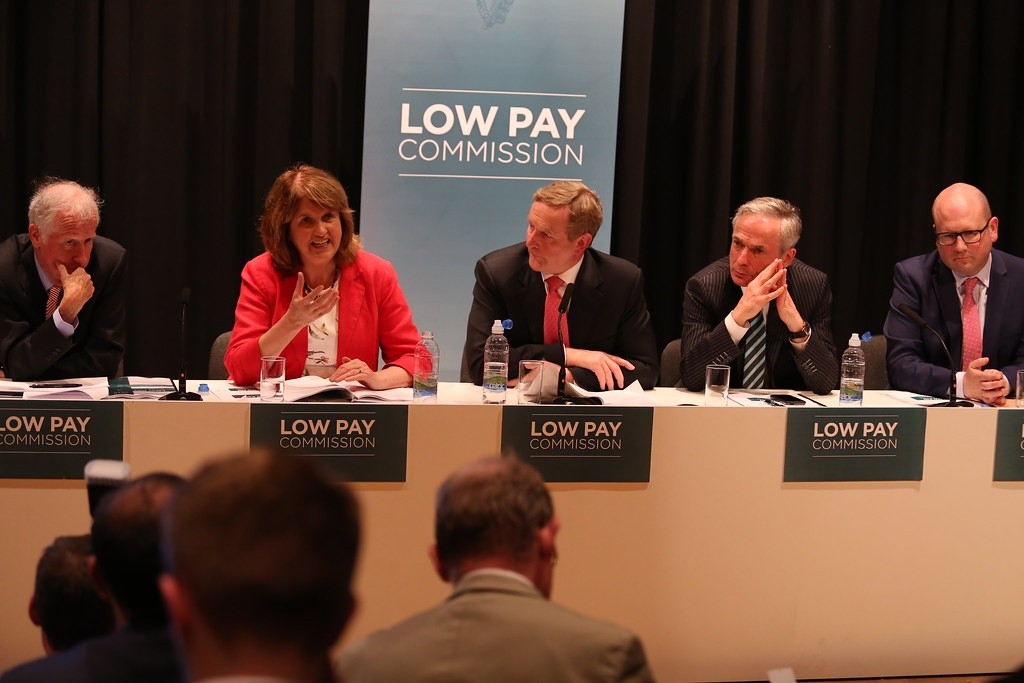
[2,377,1023,683]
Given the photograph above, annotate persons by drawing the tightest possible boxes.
[0,445,361,683]
[224,166,430,389]
[335,458,654,682]
[882,182,1024,405]
[466,181,660,389]
[681,197,837,394]
[0,182,127,382]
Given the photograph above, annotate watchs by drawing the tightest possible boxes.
[789,321,810,339]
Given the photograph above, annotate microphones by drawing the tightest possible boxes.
[541,283,581,405]
[158,288,201,400]
[898,304,960,407]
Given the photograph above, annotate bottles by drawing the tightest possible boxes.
[412,331,440,403]
[482,320,509,404]
[840,333,865,406]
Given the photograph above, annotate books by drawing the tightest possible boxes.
[103,376,178,399]
[283,375,411,401]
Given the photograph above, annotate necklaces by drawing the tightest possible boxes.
[305,265,338,291]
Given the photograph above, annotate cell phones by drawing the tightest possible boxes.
[770,394,806,405]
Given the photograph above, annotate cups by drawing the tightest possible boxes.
[260,356,285,402]
[704,365,730,407]
[1016,370,1024,407]
[517,360,544,404]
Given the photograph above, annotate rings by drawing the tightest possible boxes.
[358,368,361,372]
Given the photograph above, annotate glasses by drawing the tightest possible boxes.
[933,218,991,246]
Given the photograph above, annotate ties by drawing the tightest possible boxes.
[960,278,982,373]
[45,285,62,321]
[742,308,766,390]
[543,275,569,349]
